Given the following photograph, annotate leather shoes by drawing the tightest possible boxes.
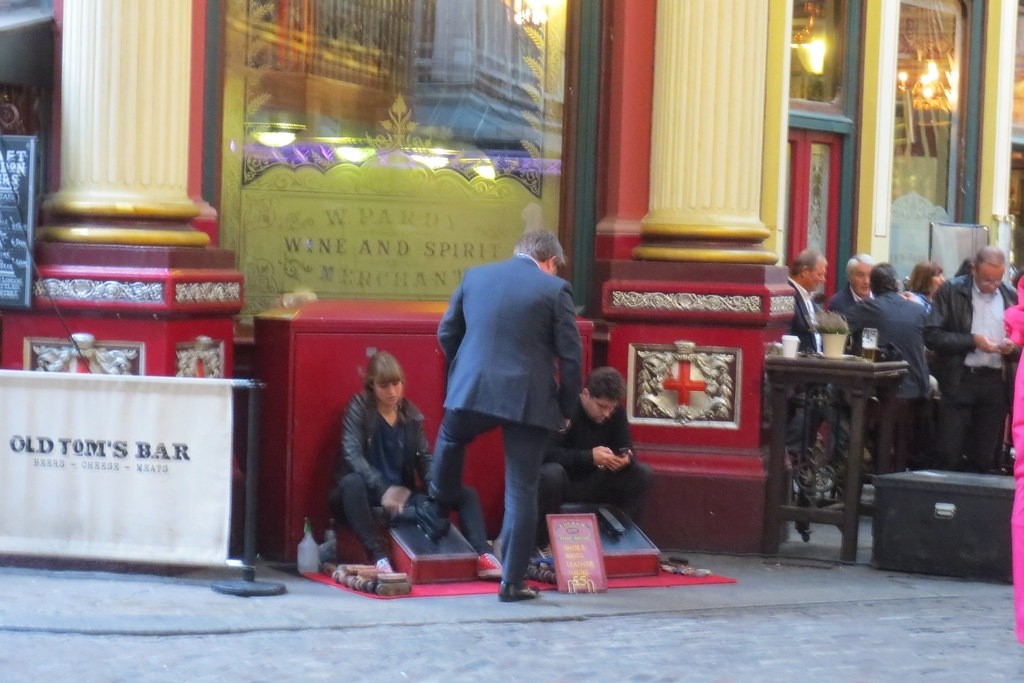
[414,497,449,539]
[499,581,539,603]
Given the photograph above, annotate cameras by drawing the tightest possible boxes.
[874,342,903,363]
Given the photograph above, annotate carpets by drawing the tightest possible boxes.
[303,569,737,600]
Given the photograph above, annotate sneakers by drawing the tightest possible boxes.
[477,553,503,578]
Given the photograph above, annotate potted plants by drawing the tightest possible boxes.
[804,307,851,356]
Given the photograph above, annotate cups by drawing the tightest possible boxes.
[781,335,799,358]
[862,327,878,363]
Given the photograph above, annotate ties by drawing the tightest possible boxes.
[807,298,822,353]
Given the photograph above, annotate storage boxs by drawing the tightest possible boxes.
[866,468,1015,586]
[560,503,663,579]
[387,518,478,582]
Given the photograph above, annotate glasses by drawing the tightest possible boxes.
[982,279,1003,287]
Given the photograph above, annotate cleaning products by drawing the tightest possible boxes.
[297,517,320,574]
[318,517,337,569]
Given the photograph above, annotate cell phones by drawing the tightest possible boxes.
[613,447,630,455]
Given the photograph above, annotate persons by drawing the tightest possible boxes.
[413,229,583,602]
[538,369,649,547]
[782,246,1022,489]
[333,351,504,578]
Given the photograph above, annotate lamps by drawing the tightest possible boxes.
[405,143,458,171]
[897,14,960,109]
[474,159,508,188]
[792,0,841,79]
[245,114,311,152]
[318,132,388,167]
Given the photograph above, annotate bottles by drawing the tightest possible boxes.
[324,518,335,541]
[296,521,318,575]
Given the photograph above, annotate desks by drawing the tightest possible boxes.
[763,349,910,566]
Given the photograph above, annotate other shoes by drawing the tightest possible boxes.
[375,557,393,572]
